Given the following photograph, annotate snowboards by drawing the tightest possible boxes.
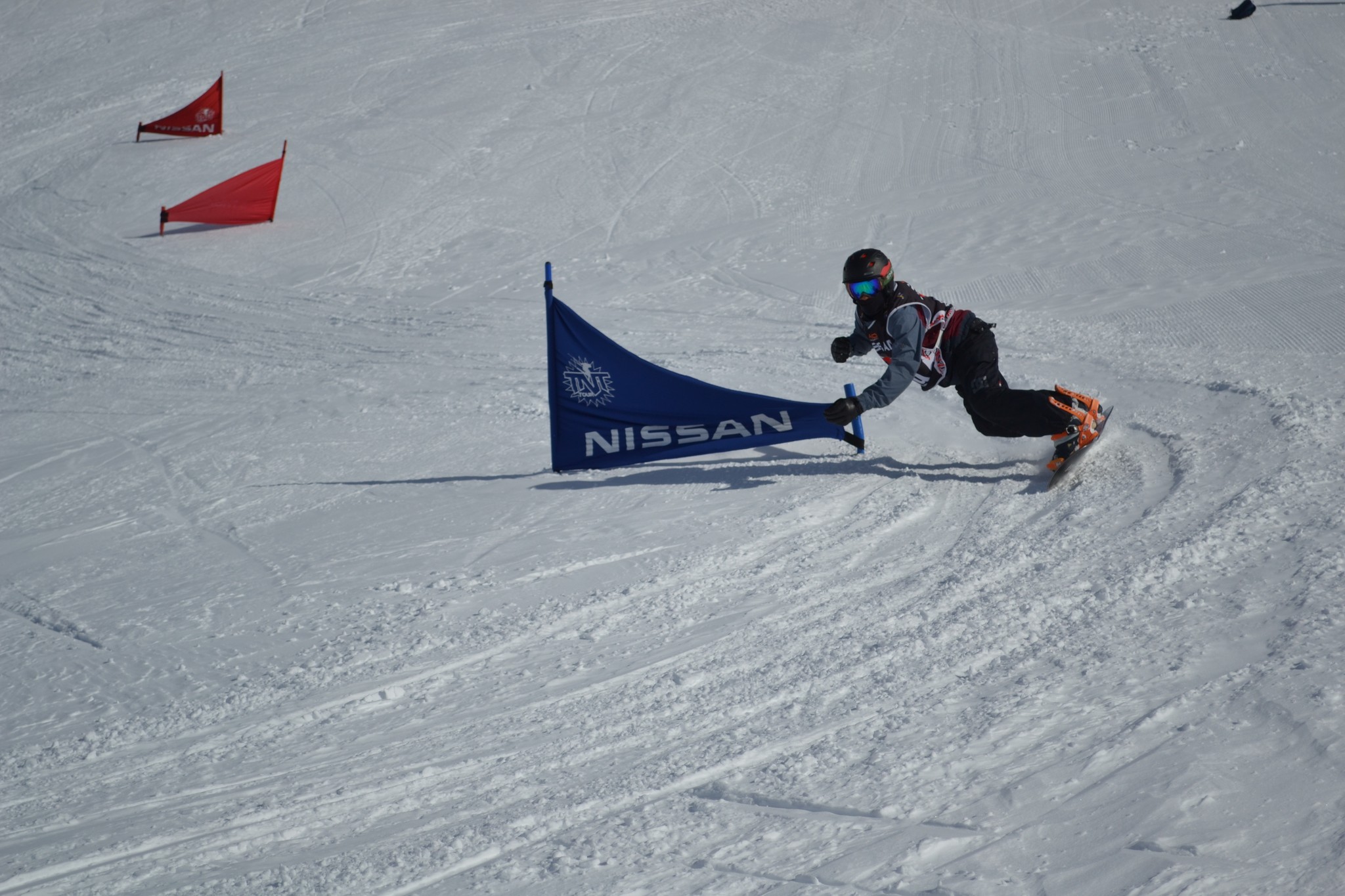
[1048,405,1114,490]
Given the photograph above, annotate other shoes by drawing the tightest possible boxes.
[1049,420,1098,471]
[1079,401,1103,418]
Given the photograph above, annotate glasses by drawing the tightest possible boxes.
[843,276,882,299]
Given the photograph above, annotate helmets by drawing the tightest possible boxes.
[843,248,894,308]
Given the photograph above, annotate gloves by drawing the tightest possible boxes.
[831,336,850,362]
[824,396,863,427]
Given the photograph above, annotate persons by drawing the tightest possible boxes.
[825,248,1102,470]
